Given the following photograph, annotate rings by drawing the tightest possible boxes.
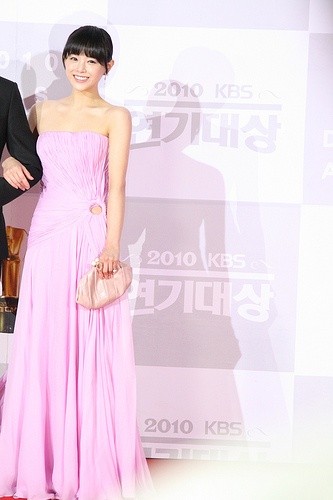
[112,269,118,272]
[94,261,100,268]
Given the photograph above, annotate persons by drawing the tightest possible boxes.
[0,26,155,500]
[0,77,43,263]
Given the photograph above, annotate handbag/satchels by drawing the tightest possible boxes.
[76,265,132,310]
